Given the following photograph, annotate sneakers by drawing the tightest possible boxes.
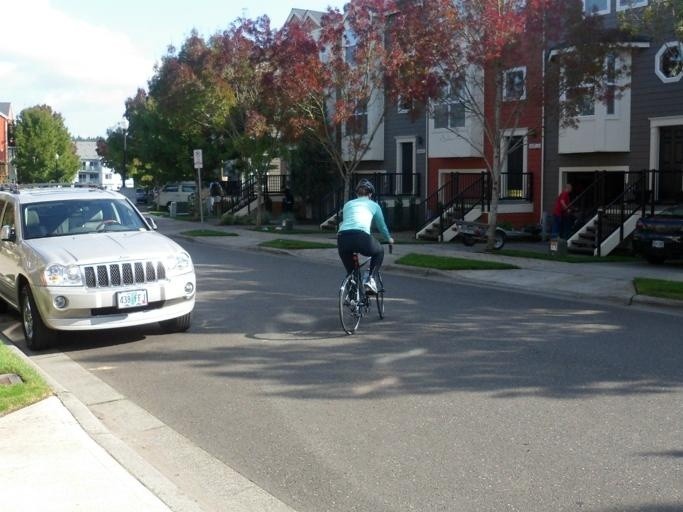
[364,278,378,294]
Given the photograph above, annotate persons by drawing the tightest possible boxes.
[202,181,213,216]
[543,182,573,256]
[209,177,224,204]
[337,176,396,310]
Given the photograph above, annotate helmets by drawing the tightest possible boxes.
[355,179,374,194]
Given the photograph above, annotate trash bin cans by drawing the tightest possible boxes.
[281,217,293,230]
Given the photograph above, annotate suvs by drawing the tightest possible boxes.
[0,179,198,353]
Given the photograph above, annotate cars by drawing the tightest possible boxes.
[631,198,682,266]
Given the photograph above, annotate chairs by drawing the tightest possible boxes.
[24,207,104,235]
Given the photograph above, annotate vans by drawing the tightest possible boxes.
[132,187,146,203]
[151,179,225,213]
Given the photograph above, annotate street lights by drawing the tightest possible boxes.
[54,151,59,181]
[120,128,128,186]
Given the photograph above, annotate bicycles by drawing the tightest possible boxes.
[334,240,393,337]
